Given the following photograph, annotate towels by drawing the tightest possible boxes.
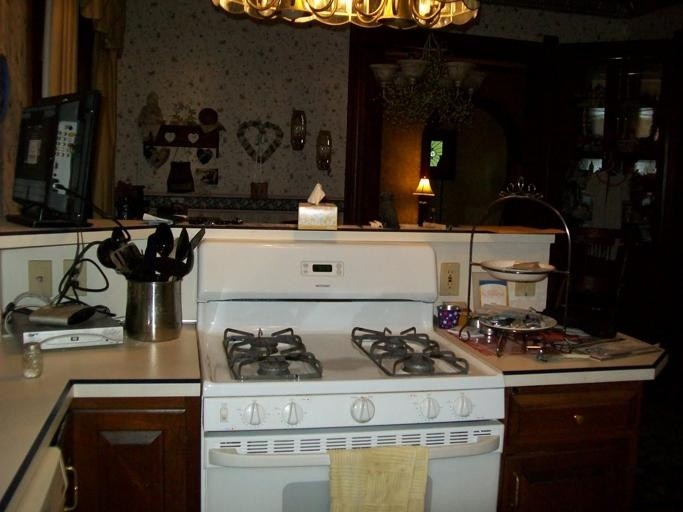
[323,445,432,511]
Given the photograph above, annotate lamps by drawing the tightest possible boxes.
[412,174,438,227]
[368,33,478,124]
[209,0,480,33]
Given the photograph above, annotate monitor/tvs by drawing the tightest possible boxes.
[6,89,103,228]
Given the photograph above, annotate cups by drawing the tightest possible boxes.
[435,305,461,328]
[121,278,182,344]
[250,181,268,196]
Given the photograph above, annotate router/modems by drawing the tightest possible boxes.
[29,302,96,328]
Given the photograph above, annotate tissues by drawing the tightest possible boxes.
[297,183,339,231]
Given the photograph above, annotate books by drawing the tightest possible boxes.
[549,324,661,363]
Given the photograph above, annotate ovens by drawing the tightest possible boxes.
[201,420,505,512]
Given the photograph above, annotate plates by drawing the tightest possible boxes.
[481,311,557,331]
[481,258,553,283]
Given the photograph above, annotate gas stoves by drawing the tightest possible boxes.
[197,329,505,433]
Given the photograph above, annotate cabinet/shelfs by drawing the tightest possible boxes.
[66,394,200,511]
[494,379,653,511]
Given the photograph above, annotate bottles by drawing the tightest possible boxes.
[22,343,44,380]
